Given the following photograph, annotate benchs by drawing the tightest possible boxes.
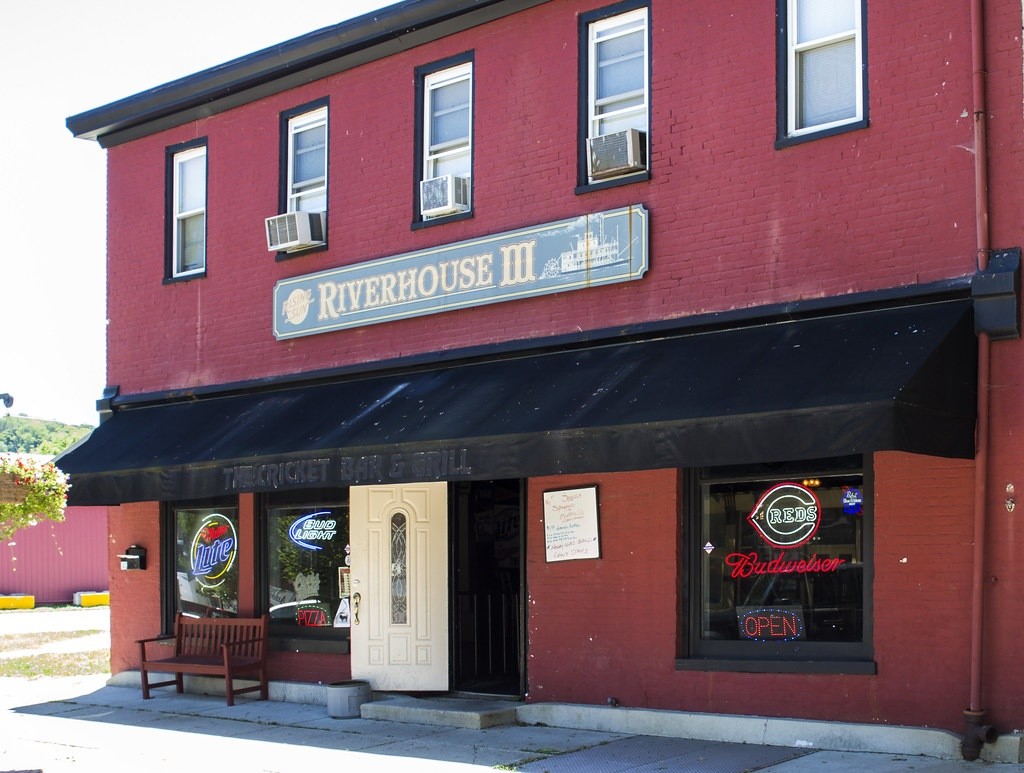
[135,611,268,706]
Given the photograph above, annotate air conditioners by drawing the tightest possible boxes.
[587,129,646,180]
[265,210,323,252]
[420,174,468,217]
